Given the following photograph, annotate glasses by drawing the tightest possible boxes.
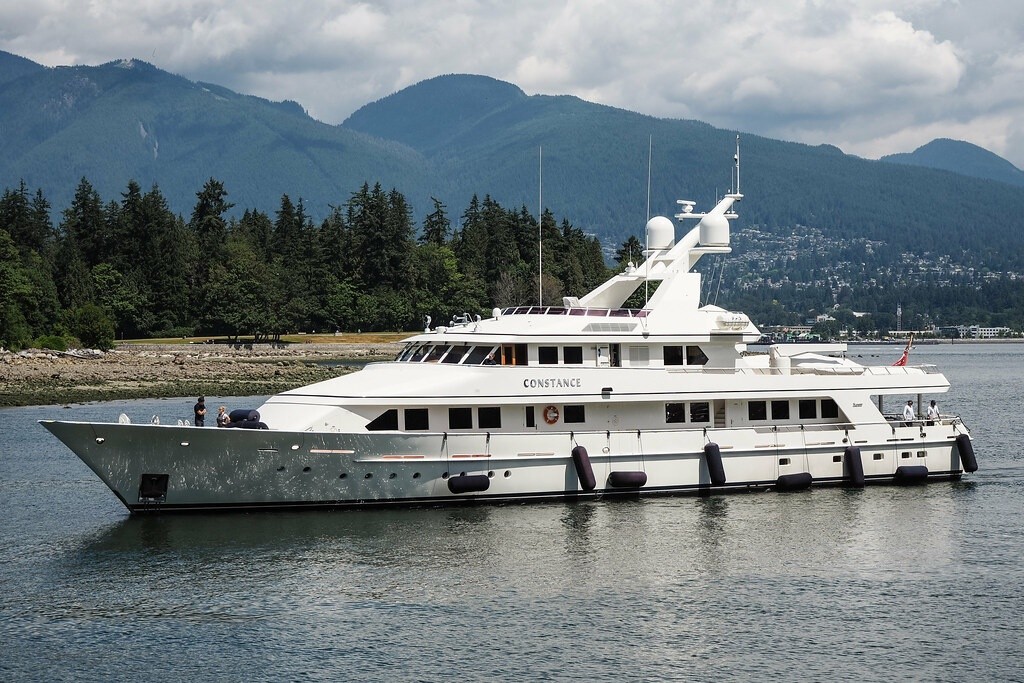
[219,409,222,410]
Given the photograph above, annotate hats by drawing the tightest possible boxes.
[931,400,936,403]
[198,396,205,402]
[907,400,913,403]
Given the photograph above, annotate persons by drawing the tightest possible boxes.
[926,400,941,426]
[217,406,231,427]
[482,353,496,365]
[194,396,206,427]
[903,400,916,427]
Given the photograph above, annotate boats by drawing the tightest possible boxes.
[35,133,978,518]
